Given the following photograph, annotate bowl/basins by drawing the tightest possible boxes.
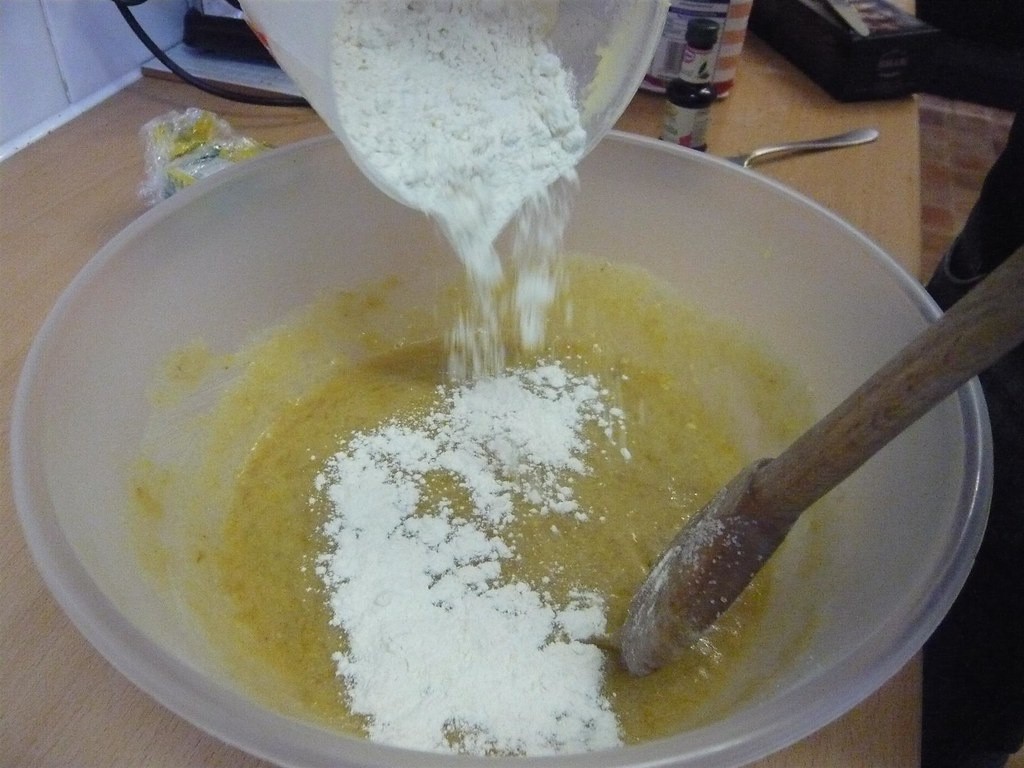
[220,1,667,206]
[9,127,991,768]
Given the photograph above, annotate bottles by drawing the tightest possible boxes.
[660,20,719,152]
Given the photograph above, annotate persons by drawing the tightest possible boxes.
[912,0,1024,768]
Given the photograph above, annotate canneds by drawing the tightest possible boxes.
[638,0,756,100]
